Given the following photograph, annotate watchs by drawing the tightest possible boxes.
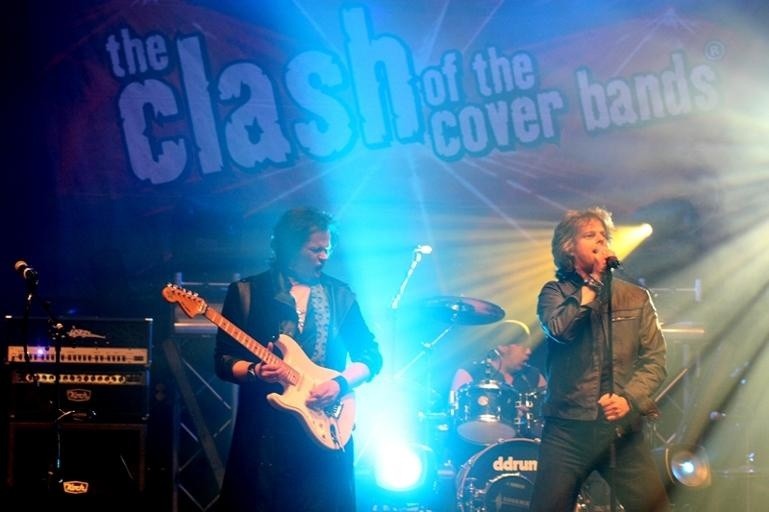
[247,364,258,385]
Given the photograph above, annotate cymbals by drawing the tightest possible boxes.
[415,294,505,325]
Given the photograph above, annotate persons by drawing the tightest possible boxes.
[527,207,667,511]
[451,320,548,393]
[213,205,382,512]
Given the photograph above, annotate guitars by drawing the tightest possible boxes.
[161,283,356,450]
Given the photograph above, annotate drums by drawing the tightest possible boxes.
[516,386,549,439]
[456,437,587,511]
[453,380,522,446]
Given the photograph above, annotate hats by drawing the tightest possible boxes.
[490,320,530,345]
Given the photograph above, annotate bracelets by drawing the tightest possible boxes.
[333,373,350,393]
[583,277,603,293]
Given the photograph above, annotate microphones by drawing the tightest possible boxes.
[15,260,38,283]
[595,249,620,269]
